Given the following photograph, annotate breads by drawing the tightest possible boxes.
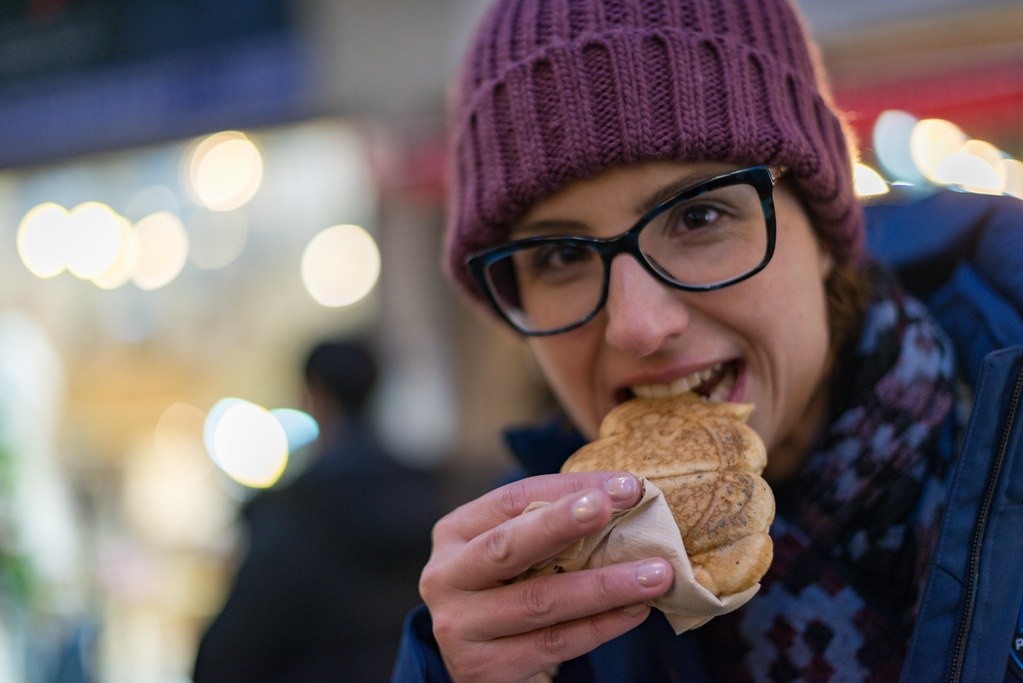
[558,392,775,600]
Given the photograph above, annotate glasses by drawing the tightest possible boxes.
[461,163,789,337]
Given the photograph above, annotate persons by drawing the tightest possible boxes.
[390,0,1023,683]
[194,340,449,683]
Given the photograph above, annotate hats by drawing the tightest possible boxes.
[442,0,862,358]
